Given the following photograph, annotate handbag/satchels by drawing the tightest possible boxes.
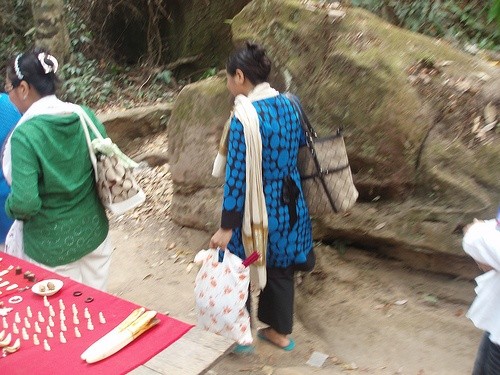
[74,104,146,215]
[193,246,253,346]
[284,91,359,216]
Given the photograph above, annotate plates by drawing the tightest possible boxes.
[31,279,63,296]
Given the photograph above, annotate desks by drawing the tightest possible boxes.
[0,251,237,375]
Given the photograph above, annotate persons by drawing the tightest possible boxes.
[6,53,109,293]
[0,94,22,253]
[210,45,310,356]
[462,216,500,375]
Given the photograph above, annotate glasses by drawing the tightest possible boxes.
[4,79,18,95]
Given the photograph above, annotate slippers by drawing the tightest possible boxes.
[257,327,295,351]
[232,344,253,356]
